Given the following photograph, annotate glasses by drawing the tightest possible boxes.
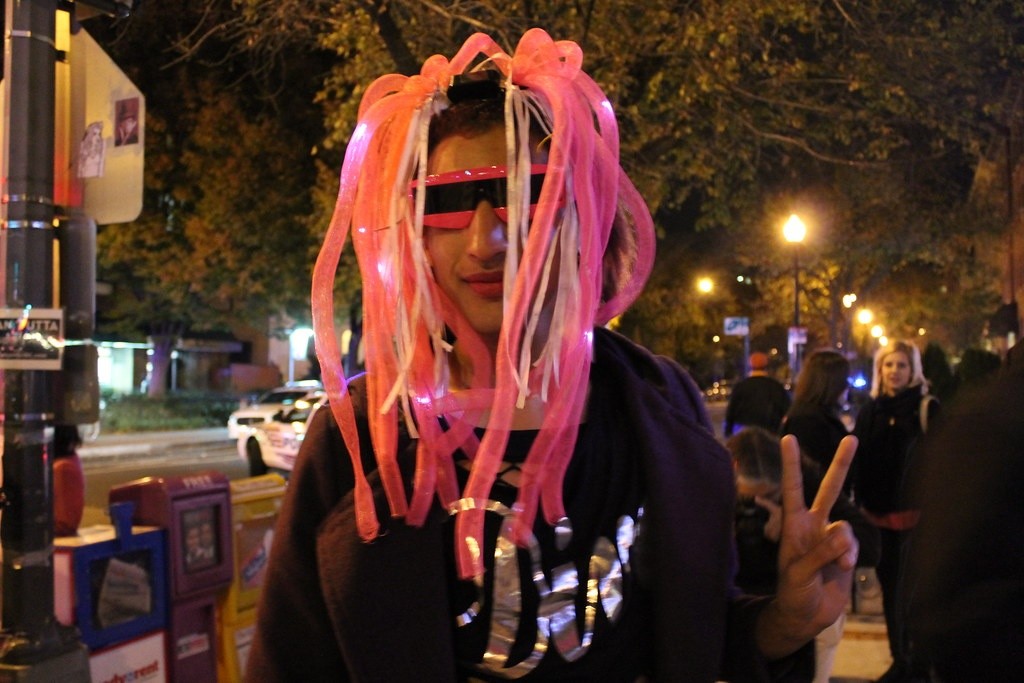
[406,163,579,229]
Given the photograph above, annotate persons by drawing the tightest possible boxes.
[186,523,216,564]
[114,100,138,146]
[246,29,857,683]
[723,337,1024,683]
[242,528,274,588]
[52,424,84,536]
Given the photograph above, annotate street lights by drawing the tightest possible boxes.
[783,214,806,375]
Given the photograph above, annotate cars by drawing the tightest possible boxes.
[226,380,322,443]
[237,387,332,478]
